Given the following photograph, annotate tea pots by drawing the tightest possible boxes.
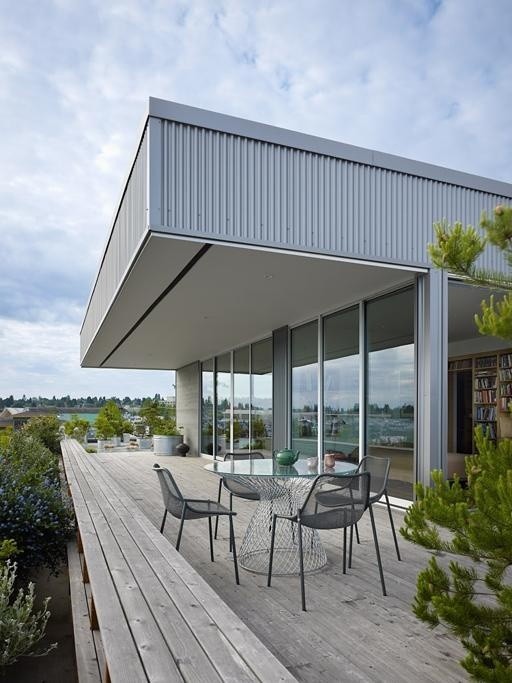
[272,448,300,466]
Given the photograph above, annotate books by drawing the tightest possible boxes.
[448,358,472,369]
[474,354,512,441]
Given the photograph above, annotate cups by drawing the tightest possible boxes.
[325,454,336,468]
[308,457,318,468]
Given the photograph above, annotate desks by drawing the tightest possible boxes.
[203,458,361,577]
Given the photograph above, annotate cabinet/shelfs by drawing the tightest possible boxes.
[448,346,511,458]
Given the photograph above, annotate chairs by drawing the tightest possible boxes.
[214,453,296,552]
[310,454,402,574]
[152,462,240,585]
[267,472,386,611]
